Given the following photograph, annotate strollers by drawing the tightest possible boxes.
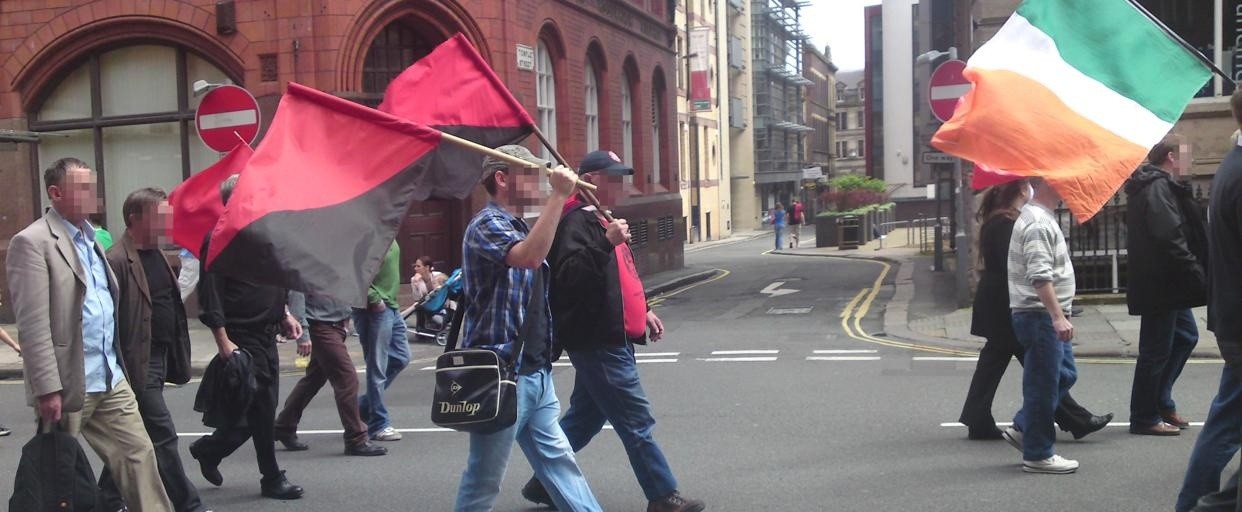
[399,268,463,346]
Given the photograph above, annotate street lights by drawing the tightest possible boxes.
[917,44,970,308]
[190,75,233,98]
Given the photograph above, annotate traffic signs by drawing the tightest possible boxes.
[922,150,957,164]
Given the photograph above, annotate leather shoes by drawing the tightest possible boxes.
[1131,413,1189,436]
[263,480,304,499]
[273,428,308,450]
[190,444,223,486]
[345,439,387,456]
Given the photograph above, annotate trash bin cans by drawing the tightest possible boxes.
[836,215,859,250]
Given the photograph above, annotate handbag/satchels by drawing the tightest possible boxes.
[429,351,517,433]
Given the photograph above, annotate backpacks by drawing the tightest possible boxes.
[8,417,101,511]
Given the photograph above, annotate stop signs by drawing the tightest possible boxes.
[928,60,971,124]
[195,83,261,155]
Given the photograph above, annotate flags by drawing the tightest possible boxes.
[973,168,1018,190]
[929,0,1214,226]
[376,31,536,200]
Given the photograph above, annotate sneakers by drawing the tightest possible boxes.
[647,493,705,512]
[522,477,557,511]
[374,426,402,440]
[968,411,1114,473]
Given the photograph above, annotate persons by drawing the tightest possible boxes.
[522,151,706,512]
[786,199,805,248]
[960,180,1113,440]
[1002,177,1078,474]
[453,145,603,512]
[770,202,787,250]
[1124,134,1209,436]
[3,81,449,512]
[1174,84,1242,512]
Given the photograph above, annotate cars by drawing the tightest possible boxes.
[762,209,772,223]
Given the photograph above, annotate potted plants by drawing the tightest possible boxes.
[815,171,897,247]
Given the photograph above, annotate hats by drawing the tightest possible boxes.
[578,150,635,177]
[480,144,551,182]
